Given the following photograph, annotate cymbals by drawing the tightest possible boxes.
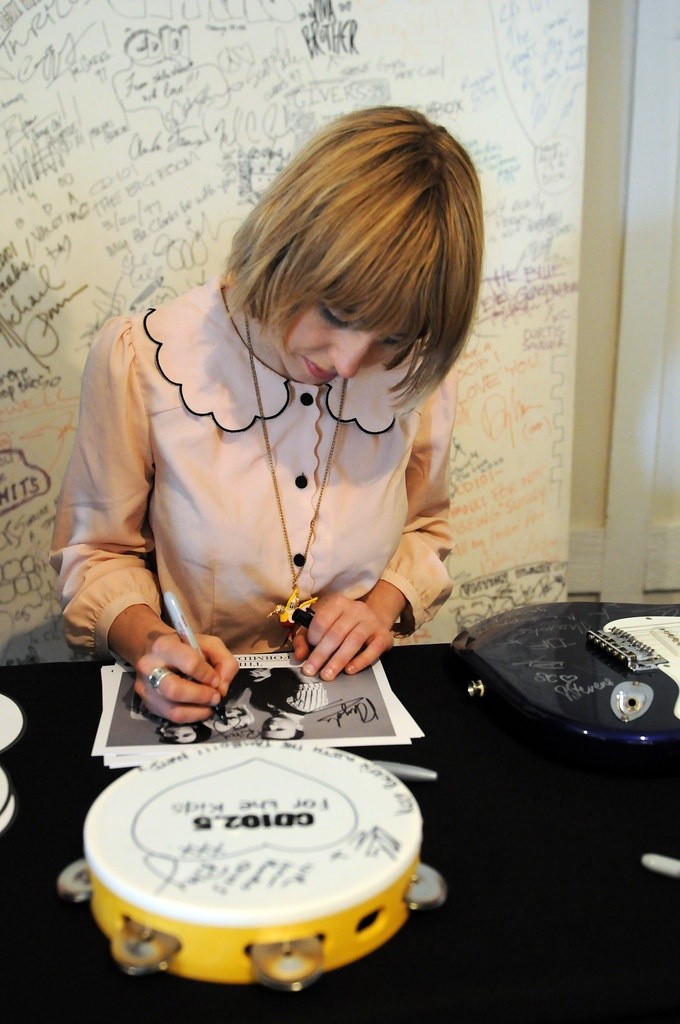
[52,841,457,1004]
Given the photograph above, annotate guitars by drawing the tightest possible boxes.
[441,592,680,779]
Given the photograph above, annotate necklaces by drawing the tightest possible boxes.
[242,303,348,623]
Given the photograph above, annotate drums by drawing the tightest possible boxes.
[82,739,426,983]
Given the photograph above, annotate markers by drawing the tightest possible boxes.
[370,760,439,784]
[164,590,230,728]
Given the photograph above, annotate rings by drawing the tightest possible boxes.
[147,665,174,692]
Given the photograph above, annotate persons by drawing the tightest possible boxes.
[50,106,486,727]
[128,667,331,743]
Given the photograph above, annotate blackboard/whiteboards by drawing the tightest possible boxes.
[1,2,592,667]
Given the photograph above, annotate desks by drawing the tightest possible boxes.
[0,639,680,1023]
